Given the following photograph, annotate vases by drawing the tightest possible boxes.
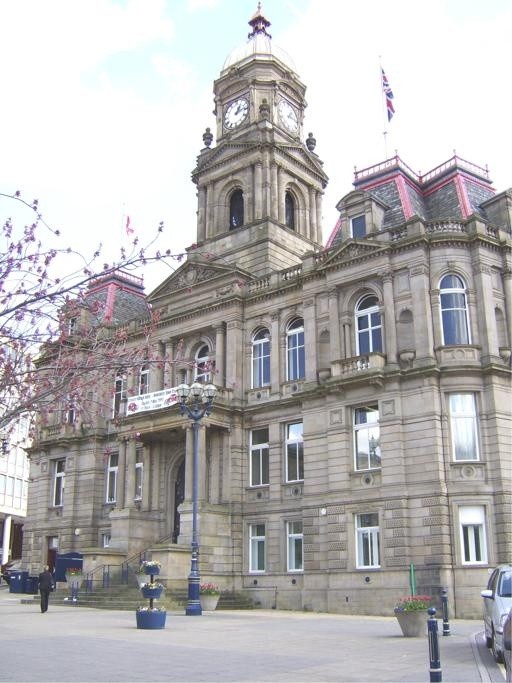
[394,610,429,637]
[201,595,221,610]
[65,576,80,582]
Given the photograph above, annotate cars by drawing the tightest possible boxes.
[0,557,22,581]
[480,564,510,665]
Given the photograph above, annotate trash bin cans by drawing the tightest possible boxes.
[10,571,39,594]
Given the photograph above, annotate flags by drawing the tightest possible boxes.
[382,68,395,123]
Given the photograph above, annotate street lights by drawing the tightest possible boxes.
[172,380,219,616]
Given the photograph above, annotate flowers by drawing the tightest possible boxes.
[64,568,81,576]
[200,583,220,594]
[392,595,432,611]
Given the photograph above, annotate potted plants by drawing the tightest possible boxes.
[136,560,166,629]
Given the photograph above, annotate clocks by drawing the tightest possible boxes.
[222,98,250,129]
[277,98,300,133]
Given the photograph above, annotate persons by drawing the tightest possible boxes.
[38,565,54,613]
